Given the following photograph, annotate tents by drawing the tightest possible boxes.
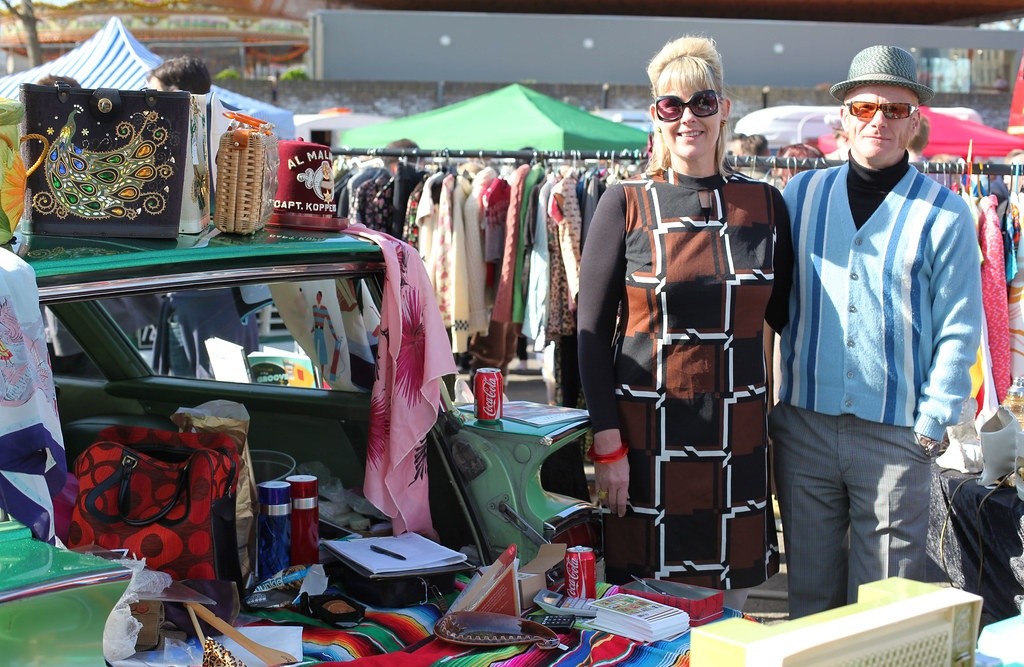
[0,15,294,140]
[818,105,1024,157]
[343,83,651,152]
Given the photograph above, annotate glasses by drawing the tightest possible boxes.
[655,90,722,123]
[843,101,918,119]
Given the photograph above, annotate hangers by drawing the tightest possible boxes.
[372,146,1024,191]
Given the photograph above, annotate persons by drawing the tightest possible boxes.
[382,139,425,174]
[45,77,163,378]
[907,113,1024,201]
[727,132,849,195]
[766,46,983,621]
[147,57,259,381]
[577,37,794,612]
[514,147,546,170]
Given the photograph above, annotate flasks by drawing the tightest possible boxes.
[255,481,292,583]
[285,475,321,566]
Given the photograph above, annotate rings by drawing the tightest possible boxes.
[598,490,608,500]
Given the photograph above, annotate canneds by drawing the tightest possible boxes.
[565,545,597,599]
[473,368,503,421]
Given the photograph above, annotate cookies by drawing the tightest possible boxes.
[321,600,357,614]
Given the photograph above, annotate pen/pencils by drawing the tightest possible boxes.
[370,544,407,560]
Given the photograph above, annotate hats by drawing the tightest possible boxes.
[266,137,349,231]
[830,45,935,107]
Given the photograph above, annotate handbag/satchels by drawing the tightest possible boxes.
[0,97,48,249]
[340,563,457,608]
[18,82,190,239]
[214,112,279,234]
[68,426,252,618]
[0,248,67,543]
[171,399,261,592]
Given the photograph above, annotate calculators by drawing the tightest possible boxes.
[532,588,599,617]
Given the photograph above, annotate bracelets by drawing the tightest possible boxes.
[587,443,629,463]
